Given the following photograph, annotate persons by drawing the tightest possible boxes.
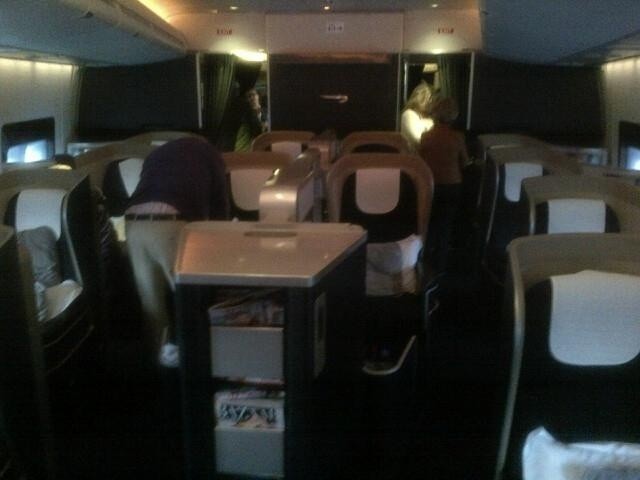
[415,99,471,254]
[216,86,253,154]
[120,126,236,366]
[400,78,435,144]
[243,86,271,137]
[49,152,126,296]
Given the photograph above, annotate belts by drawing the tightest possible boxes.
[124,213,183,221]
